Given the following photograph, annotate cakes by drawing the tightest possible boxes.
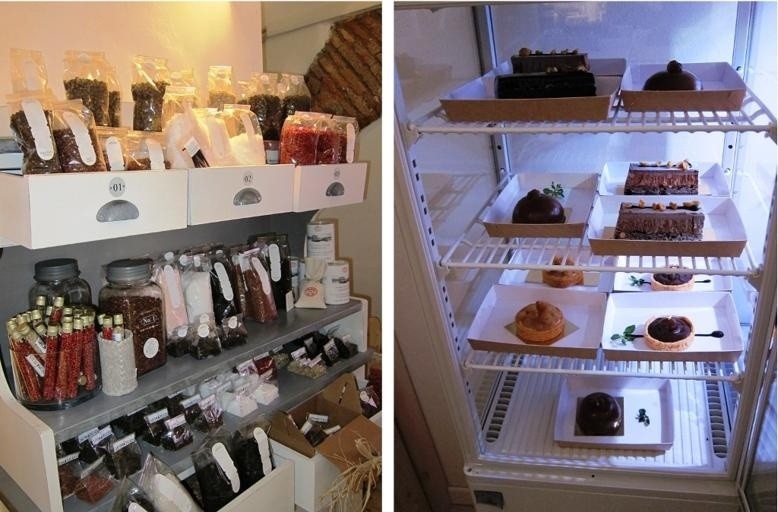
[492,66,597,99]
[543,255,583,287]
[511,189,566,224]
[642,61,703,91]
[651,265,694,291]
[511,47,591,73]
[643,314,695,352]
[576,392,623,436]
[614,198,705,240]
[623,158,699,194]
[514,300,565,342]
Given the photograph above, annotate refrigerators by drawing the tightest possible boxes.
[394,3,778,510]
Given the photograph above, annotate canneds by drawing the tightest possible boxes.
[288,218,351,306]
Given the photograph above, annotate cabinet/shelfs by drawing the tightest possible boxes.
[1,160,370,512]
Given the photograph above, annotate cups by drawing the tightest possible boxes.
[7,343,102,412]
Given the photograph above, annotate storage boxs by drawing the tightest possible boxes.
[262,371,381,511]
